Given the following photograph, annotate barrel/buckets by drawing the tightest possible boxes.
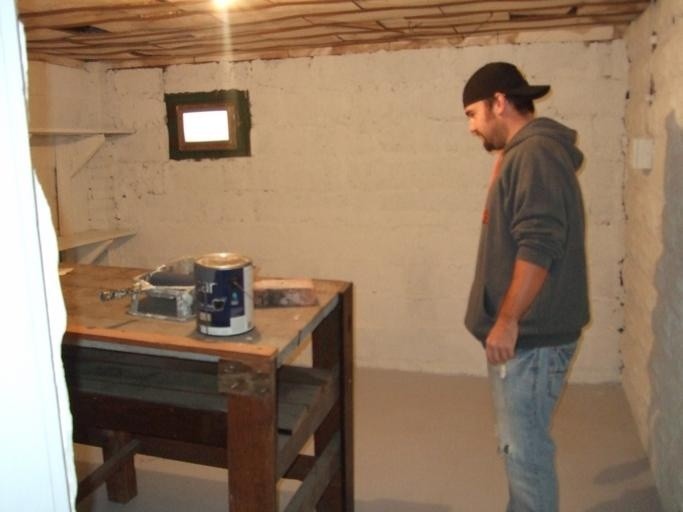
[194,254,256,337]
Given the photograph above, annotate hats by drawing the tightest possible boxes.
[462,62,551,109]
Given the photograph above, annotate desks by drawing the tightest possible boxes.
[59,261,355,511]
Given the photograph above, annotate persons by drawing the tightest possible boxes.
[460,58,593,511]
